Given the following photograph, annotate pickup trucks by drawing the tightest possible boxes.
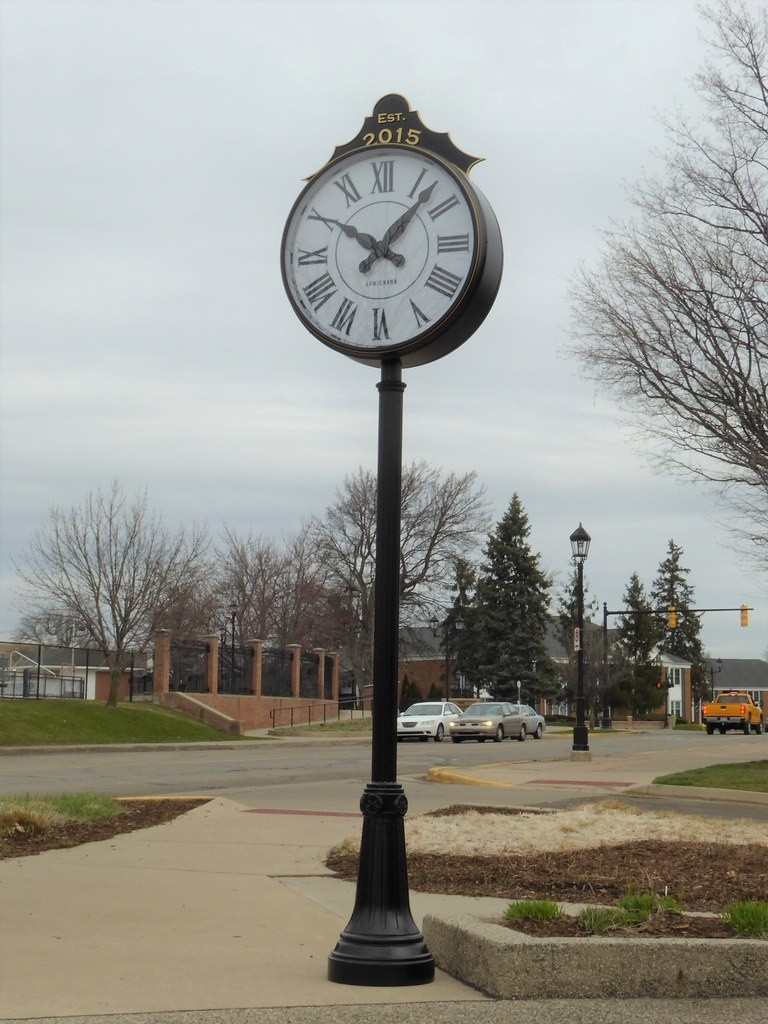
[701,690,765,735]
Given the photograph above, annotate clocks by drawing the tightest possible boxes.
[280,94,505,371]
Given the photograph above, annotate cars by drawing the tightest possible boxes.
[448,701,528,743]
[139,671,186,694]
[487,704,547,740]
[396,702,464,743]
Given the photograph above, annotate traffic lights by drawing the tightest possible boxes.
[656,681,664,689]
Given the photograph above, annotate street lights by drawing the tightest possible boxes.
[229,600,240,694]
[569,521,592,751]
[710,657,722,702]
[445,614,465,703]
